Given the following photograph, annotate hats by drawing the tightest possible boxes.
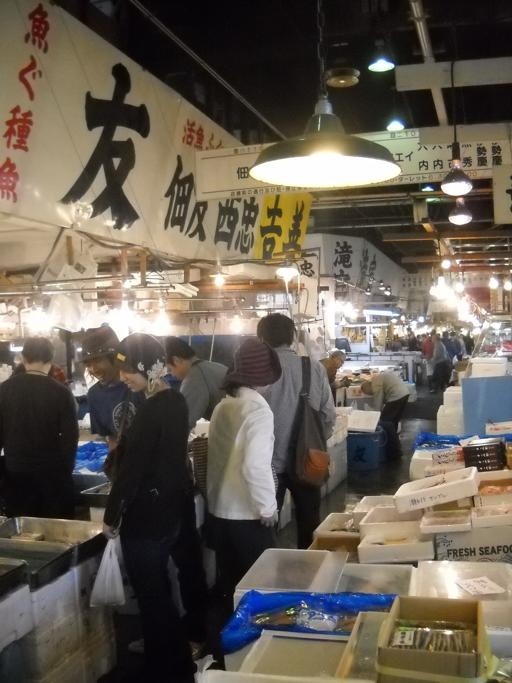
[77,325,122,365]
[223,335,282,387]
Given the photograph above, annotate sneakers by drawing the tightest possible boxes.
[190,640,207,661]
[128,638,145,654]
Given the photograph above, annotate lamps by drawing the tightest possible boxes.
[448,197,472,226]
[248,0,402,189]
[441,63,474,196]
[368,1,396,73]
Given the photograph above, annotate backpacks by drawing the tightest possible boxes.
[285,355,331,489]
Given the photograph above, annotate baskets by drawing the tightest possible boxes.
[462,441,507,473]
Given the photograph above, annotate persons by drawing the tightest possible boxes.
[362,373,406,462]
[159,336,234,433]
[205,339,311,635]
[68,325,145,443]
[1,338,78,518]
[228,315,336,554]
[102,333,219,672]
[430,334,452,393]
[405,330,476,369]
[319,350,345,386]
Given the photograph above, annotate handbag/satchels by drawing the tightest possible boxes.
[103,448,117,483]
[190,434,281,505]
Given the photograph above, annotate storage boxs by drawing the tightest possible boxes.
[443,386,462,405]
[437,405,464,435]
[0,517,112,683]
[325,414,348,448]
[472,358,507,377]
